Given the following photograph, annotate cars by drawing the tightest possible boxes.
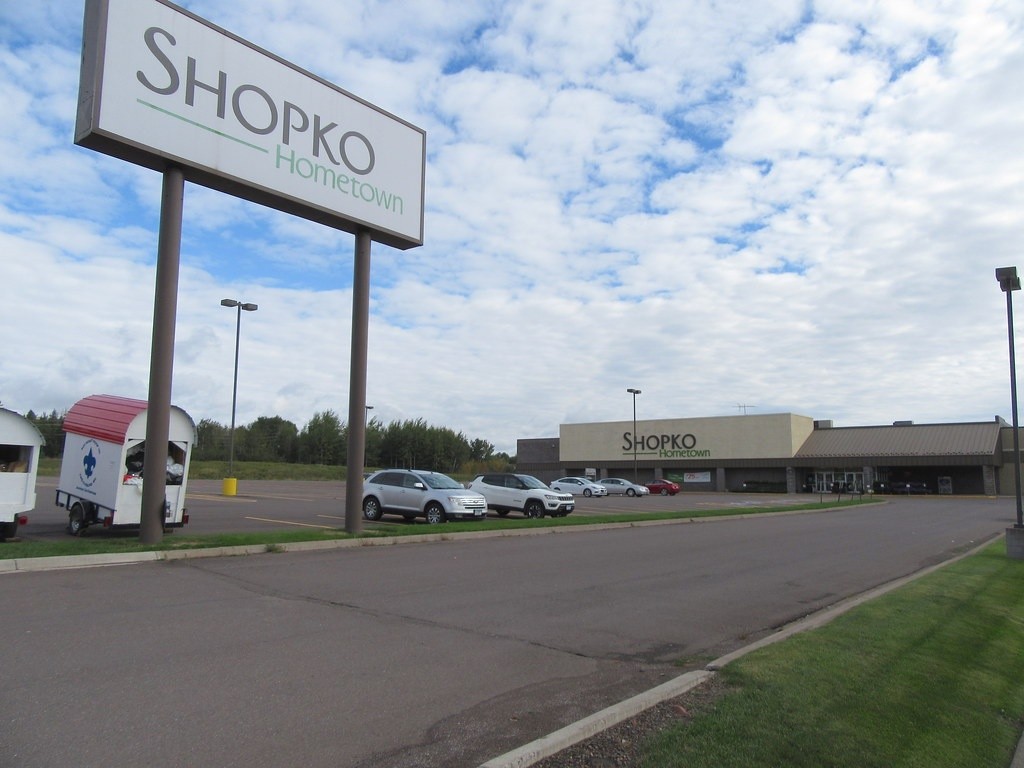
[362,467,488,522]
[467,472,575,518]
[639,478,680,496]
[549,476,608,498]
[594,478,651,497]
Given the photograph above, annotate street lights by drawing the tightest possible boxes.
[220,298,258,495]
[996,266,1024,563]
[365,405,374,467]
[626,388,642,485]
